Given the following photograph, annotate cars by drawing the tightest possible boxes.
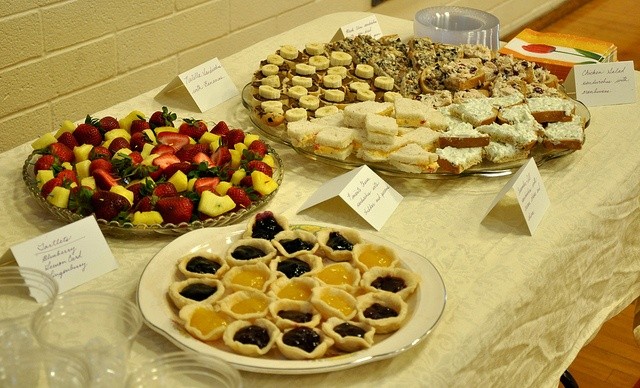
[0,346,93,388]
[0,265,59,388]
[123,351,243,388]
[31,290,145,388]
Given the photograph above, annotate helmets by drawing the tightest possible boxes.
[136,219,448,376]
[22,117,285,238]
[240,81,592,181]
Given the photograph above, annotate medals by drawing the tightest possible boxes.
[282,88,445,176]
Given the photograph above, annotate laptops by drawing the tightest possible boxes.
[437,88,590,177]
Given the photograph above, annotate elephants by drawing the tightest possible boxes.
[33,114,272,224]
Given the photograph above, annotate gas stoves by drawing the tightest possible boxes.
[250,39,402,128]
[332,34,564,104]
